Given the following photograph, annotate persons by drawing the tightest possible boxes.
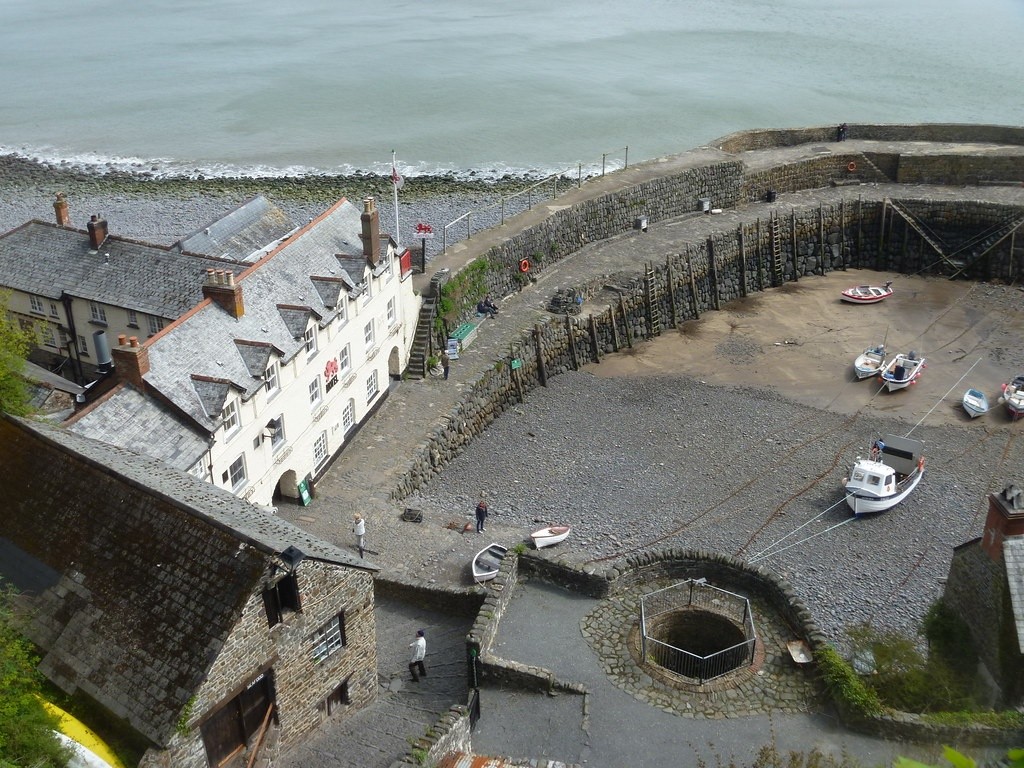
[351,512,366,548]
[835,123,848,142]
[477,296,500,319]
[440,349,451,383]
[475,500,489,534]
[407,629,427,682]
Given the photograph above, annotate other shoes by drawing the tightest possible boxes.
[482,528,486,531]
[479,531,483,533]
[410,678,419,682]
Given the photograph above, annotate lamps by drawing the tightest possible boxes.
[274,544,307,577]
[262,419,280,442]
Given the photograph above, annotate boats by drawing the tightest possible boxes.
[962,388,989,419]
[843,434,926,516]
[530,525,571,550]
[854,345,887,380]
[880,351,926,392]
[471,543,509,583]
[841,281,894,304]
[997,376,1024,420]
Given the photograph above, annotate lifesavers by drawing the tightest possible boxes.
[847,161,857,172]
[520,260,529,272]
[918,456,926,471]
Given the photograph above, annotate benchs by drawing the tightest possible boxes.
[477,557,500,570]
[488,548,505,557]
